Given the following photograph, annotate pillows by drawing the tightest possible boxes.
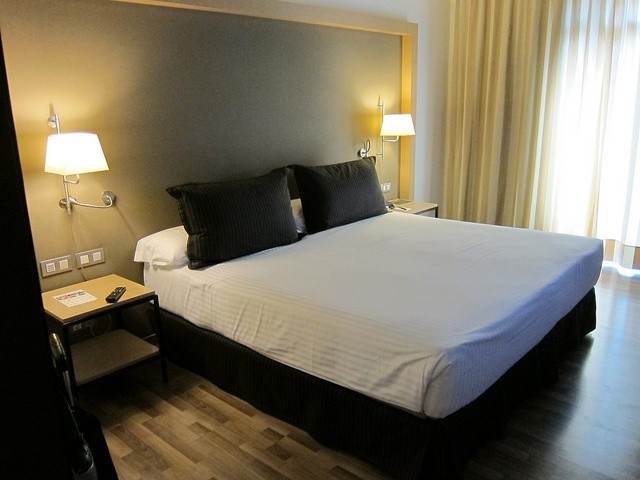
[165,168,306,270]
[289,156,388,235]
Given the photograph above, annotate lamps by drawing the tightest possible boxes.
[360,101,416,160]
[45,113,116,215]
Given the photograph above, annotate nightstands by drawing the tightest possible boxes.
[43,274,170,415]
[386,199,439,219]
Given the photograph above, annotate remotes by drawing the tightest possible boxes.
[104,285,128,303]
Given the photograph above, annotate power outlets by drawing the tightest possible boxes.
[40,255,74,279]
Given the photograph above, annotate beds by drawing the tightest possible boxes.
[133,192,603,471]
